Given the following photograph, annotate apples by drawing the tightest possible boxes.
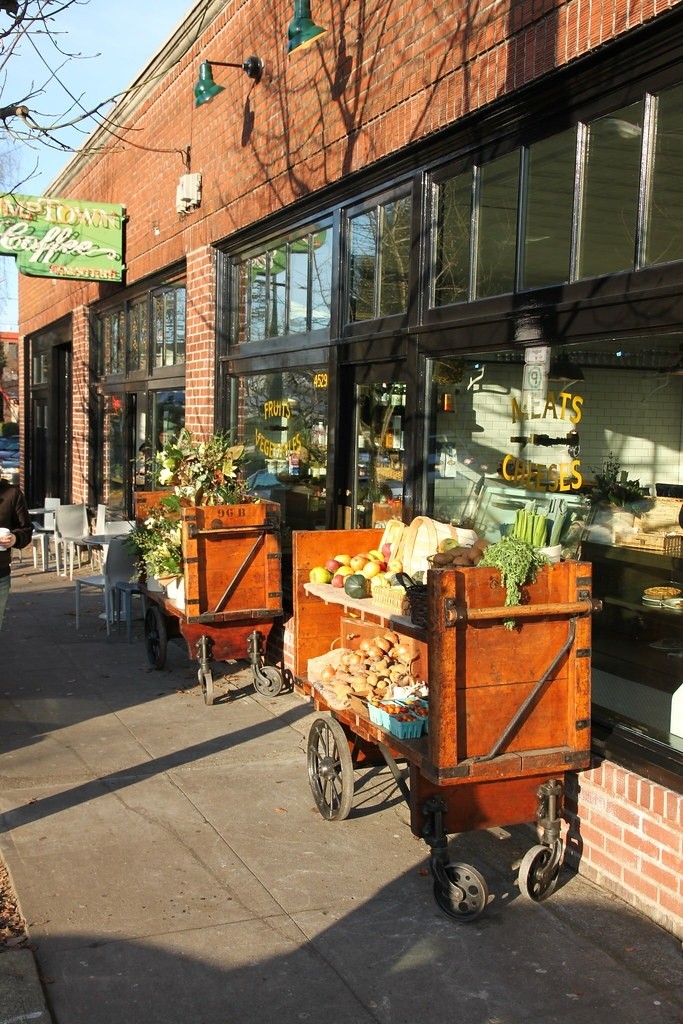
[440,538,460,552]
[309,543,402,588]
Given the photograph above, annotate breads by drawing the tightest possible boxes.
[644,587,681,600]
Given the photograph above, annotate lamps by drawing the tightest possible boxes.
[546,366,586,382]
[195,54,264,109]
[653,365,683,376]
[286,0,327,56]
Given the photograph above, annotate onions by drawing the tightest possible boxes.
[321,633,411,682]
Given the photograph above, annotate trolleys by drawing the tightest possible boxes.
[291,526,603,924]
[133,491,285,706]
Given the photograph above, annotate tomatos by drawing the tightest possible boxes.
[369,699,428,722]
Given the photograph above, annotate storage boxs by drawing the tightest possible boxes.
[348,690,430,740]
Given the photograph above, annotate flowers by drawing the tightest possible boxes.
[112,427,261,587]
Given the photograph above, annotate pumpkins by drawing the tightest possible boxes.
[344,570,425,599]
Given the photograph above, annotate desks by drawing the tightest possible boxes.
[606,581,683,626]
[82,534,143,623]
[28,508,70,572]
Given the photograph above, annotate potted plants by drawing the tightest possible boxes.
[587,452,657,536]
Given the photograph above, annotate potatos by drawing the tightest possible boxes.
[432,540,490,570]
[335,670,402,700]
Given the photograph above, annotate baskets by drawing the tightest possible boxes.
[368,696,429,739]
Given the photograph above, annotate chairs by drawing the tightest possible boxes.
[12,497,146,640]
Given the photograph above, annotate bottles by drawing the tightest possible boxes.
[312,461,320,482]
[277,459,289,477]
[267,459,277,475]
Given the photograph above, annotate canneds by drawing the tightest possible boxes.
[267,460,289,476]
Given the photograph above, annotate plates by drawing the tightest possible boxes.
[662,598,683,610]
[642,596,664,602]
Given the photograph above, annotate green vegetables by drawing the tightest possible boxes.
[477,535,553,631]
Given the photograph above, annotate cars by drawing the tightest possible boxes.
[245,469,297,500]
[0,434,20,486]
[428,434,481,486]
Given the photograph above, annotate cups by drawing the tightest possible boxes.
[0,528,10,551]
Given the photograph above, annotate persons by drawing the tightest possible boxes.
[139,442,161,475]
[156,429,177,453]
[0,469,33,630]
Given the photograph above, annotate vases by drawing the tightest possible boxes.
[147,573,185,610]
